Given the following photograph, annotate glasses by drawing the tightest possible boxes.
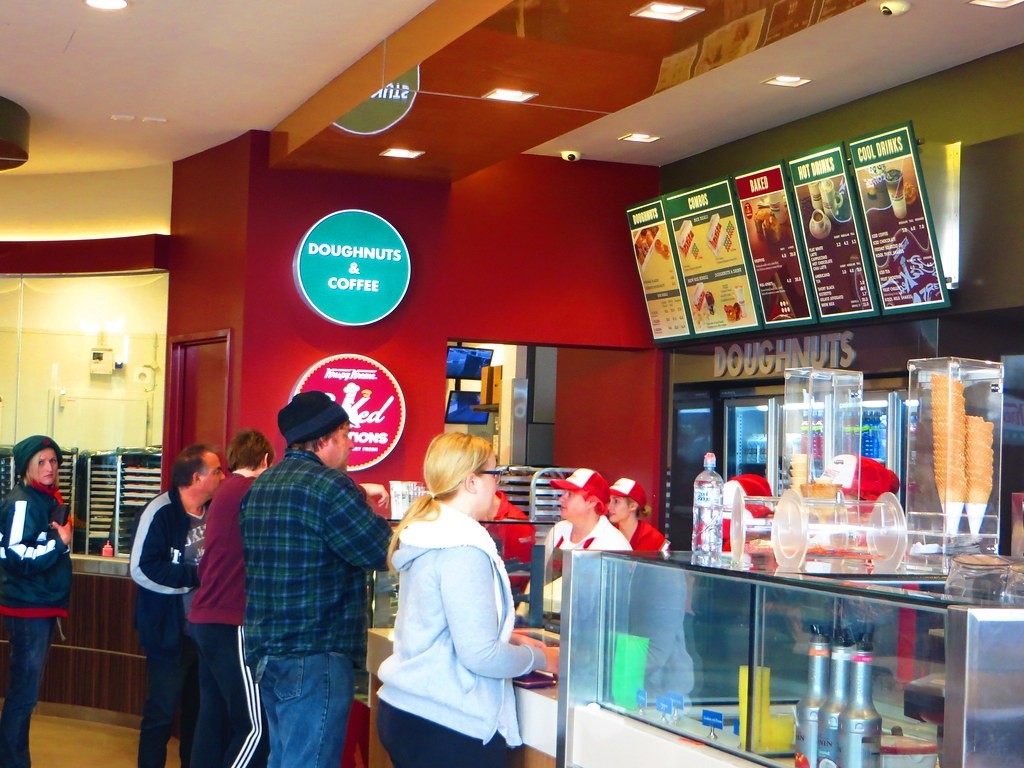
[474,469,503,484]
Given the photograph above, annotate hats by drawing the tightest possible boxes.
[821,453,900,504]
[549,467,612,516]
[277,391,347,448]
[722,474,775,519]
[13,435,63,477]
[608,477,646,509]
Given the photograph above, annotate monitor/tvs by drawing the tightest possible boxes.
[444,390,489,425]
[445,346,494,380]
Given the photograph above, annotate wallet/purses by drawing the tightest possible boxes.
[47,504,71,540]
[512,670,557,689]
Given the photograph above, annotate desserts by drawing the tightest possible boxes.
[754,210,781,243]
[904,182,917,204]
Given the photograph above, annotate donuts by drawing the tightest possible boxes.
[724,302,742,322]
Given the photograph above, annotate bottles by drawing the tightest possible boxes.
[795,624,831,768]
[817,628,856,768]
[801,408,886,458]
[691,452,725,557]
[102,540,113,557]
[837,632,882,768]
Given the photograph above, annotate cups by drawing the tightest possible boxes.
[808,182,823,210]
[885,169,907,219]
[812,209,826,233]
[612,632,649,710]
[819,179,843,217]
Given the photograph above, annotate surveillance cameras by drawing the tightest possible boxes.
[561,151,581,162]
[879,1,912,17]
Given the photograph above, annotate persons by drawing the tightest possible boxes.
[374,431,559,768]
[185,430,390,768]
[0,435,74,768]
[513,468,634,618]
[479,488,536,609]
[240,392,398,768]
[690,472,801,700]
[607,476,693,551]
[785,453,918,695]
[127,445,225,768]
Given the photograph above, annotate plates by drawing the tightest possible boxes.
[809,214,831,239]
